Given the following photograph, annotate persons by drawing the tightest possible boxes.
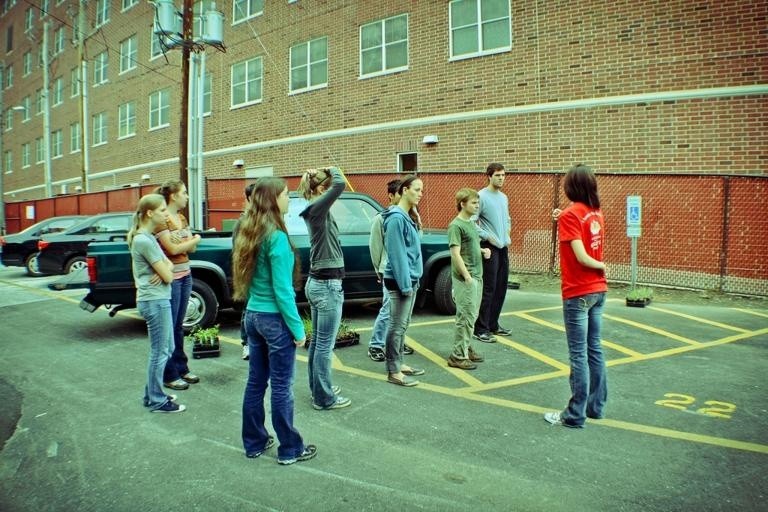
[368,180,423,362]
[543,163,609,430]
[127,194,186,414]
[231,183,257,360]
[298,165,353,411]
[153,178,201,391]
[381,174,424,386]
[470,162,512,343]
[234,176,318,466]
[446,188,492,370]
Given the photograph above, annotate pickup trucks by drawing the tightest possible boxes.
[47,190,457,336]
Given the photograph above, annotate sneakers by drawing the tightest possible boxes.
[181,373,199,383]
[448,356,477,370]
[386,374,419,387]
[400,365,425,376]
[150,401,186,413]
[368,346,387,361]
[162,377,189,390]
[544,411,586,426]
[403,344,414,354]
[142,394,178,407]
[473,332,497,343]
[468,346,484,361]
[311,384,352,410]
[246,435,274,458]
[277,444,318,465]
[491,325,513,336]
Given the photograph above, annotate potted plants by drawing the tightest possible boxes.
[187,323,220,359]
[301,311,360,349]
[625,286,654,308]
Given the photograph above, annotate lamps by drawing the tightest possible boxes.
[233,159,244,168]
[421,135,438,145]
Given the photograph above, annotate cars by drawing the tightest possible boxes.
[36,212,137,275]
[0,215,95,277]
